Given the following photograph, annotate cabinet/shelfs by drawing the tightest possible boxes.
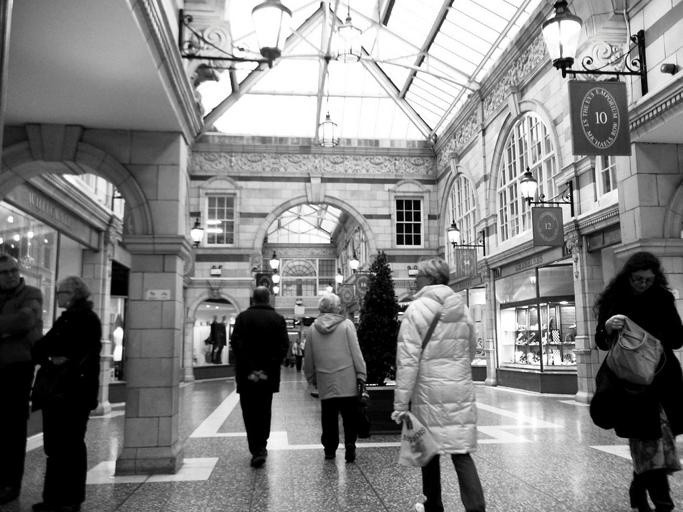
[514,301,577,366]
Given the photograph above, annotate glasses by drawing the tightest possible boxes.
[56,291,70,298]
[631,276,654,287]
[417,273,429,280]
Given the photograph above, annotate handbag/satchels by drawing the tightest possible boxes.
[605,316,667,387]
[356,379,372,439]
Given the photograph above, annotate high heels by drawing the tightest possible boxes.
[628,475,650,511]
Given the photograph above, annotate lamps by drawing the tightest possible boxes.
[252,250,281,296]
[326,251,369,296]
[318,56,339,147]
[178,0,292,69]
[540,0,648,97]
[448,218,485,256]
[190,218,204,248]
[337,0,362,65]
[520,166,574,217]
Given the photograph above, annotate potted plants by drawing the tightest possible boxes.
[356,249,412,436]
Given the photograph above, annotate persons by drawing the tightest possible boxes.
[207,313,219,362]
[303,291,369,463]
[0,252,43,511]
[392,256,487,512]
[111,325,126,361]
[29,273,102,511]
[214,315,227,363]
[588,250,682,512]
[292,337,305,373]
[229,286,289,466]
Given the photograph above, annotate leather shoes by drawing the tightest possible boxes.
[415,501,425,511]
[0,486,80,512]
